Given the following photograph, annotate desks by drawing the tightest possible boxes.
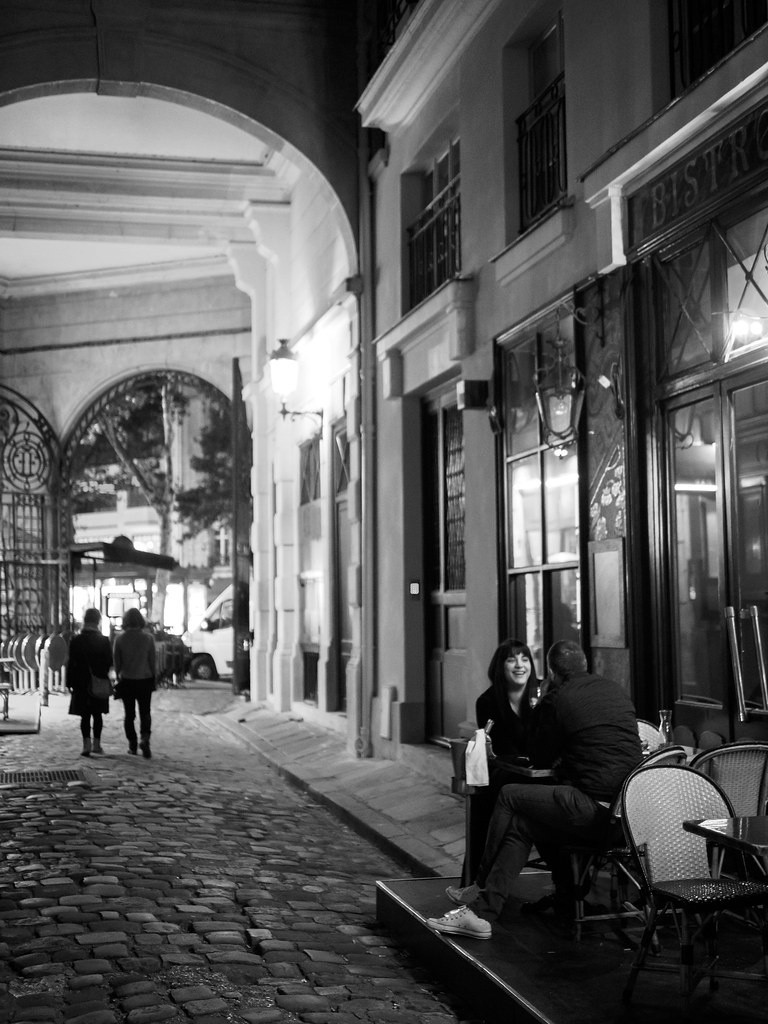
[683,816,768,881]
[494,757,555,777]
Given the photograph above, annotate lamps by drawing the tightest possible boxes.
[270,338,324,440]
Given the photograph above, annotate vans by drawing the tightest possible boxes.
[180,581,253,681]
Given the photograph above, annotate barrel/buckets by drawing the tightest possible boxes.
[432,736,492,780]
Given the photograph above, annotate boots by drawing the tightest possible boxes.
[94,738,103,752]
[80,738,92,756]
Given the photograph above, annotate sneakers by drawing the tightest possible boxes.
[428,905,493,940]
[446,882,486,906]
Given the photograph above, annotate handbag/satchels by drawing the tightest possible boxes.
[92,675,112,699]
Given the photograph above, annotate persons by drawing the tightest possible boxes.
[113,607,156,760]
[65,608,113,756]
[426,638,645,940]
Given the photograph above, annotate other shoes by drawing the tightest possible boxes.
[128,750,137,755]
[140,741,152,758]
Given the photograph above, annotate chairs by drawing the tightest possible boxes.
[569,718,768,1019]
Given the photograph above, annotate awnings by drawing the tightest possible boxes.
[69,536,179,571]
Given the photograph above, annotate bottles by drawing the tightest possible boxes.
[465,720,494,741]
[660,710,674,749]
